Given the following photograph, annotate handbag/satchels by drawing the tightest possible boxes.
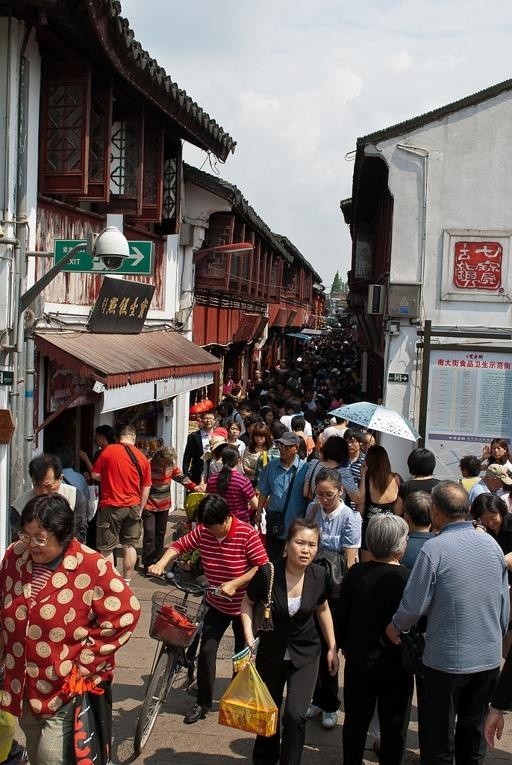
[152,605,197,647]
[72,678,113,764]
[400,627,429,675]
[265,511,285,537]
[253,561,275,636]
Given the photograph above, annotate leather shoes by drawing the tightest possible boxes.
[183,703,208,723]
[373,739,381,757]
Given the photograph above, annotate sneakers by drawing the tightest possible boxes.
[3,741,29,765]
[322,708,339,728]
[305,703,322,717]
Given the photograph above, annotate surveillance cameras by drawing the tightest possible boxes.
[94,226,130,271]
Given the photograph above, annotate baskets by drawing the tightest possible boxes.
[148,591,209,647]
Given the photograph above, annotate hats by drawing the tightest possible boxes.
[208,426,228,439]
[201,435,240,459]
[274,431,301,446]
[487,463,512,484]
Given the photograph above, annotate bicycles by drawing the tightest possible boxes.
[132,566,236,757]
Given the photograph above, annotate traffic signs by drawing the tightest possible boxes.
[52,240,155,276]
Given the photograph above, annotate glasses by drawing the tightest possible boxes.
[275,443,296,449]
[202,417,216,422]
[313,486,339,499]
[18,531,56,547]
[33,478,57,490]
